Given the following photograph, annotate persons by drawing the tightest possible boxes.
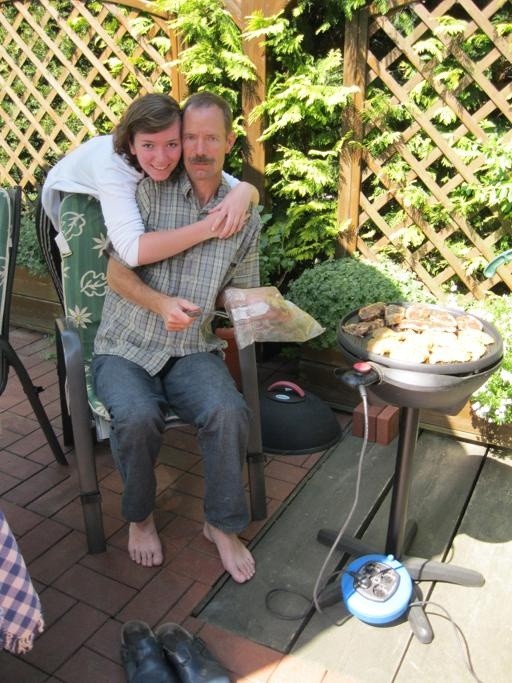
[38,92,262,316]
[90,93,262,584]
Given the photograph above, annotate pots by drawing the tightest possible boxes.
[336,305,503,412]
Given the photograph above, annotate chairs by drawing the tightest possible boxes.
[36,190,268,554]
[1,184,70,464]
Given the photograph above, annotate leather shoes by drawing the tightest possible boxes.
[120,618,177,682]
[153,623,232,682]
